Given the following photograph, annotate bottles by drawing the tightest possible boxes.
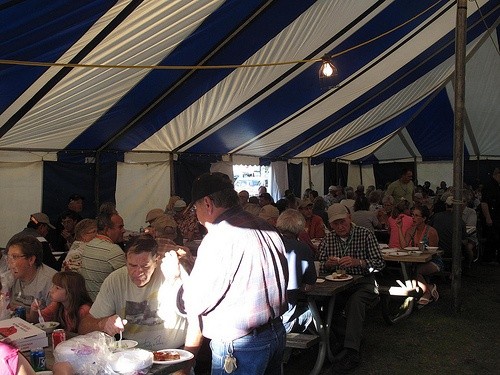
[140,224,145,234]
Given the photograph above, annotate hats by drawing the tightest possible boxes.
[150,214,178,233]
[328,185,337,192]
[184,172,234,214]
[327,202,351,224]
[30,212,56,230]
[145,208,164,224]
[297,199,314,211]
[414,192,423,199]
[259,204,280,221]
[173,200,187,209]
[283,189,292,197]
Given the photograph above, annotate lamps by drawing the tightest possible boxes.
[318,54,339,81]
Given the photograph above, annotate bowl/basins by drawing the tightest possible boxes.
[35,322,60,334]
[193,240,201,245]
[426,247,439,252]
[183,239,189,244]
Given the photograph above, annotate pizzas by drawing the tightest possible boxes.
[152,351,180,361]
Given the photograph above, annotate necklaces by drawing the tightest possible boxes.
[338,228,354,257]
[19,270,37,290]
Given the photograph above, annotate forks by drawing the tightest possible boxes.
[35,298,44,326]
[117,332,122,348]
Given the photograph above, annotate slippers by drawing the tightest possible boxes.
[417,296,433,305]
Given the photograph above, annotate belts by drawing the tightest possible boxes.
[247,315,282,336]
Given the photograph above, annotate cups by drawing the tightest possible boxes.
[314,261,320,277]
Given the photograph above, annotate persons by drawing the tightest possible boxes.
[0,167,208,375]
[160,172,289,375]
[238,168,500,367]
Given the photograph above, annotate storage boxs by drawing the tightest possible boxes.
[0,317,49,352]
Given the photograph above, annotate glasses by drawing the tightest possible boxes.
[411,213,423,218]
[6,255,25,265]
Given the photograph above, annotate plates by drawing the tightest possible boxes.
[389,252,409,256]
[316,279,325,283]
[153,349,195,365]
[36,371,53,375]
[54,338,154,375]
[408,250,423,256]
[404,246,420,250]
[325,275,354,282]
[382,248,401,253]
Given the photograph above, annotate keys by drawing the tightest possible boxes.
[223,341,237,374]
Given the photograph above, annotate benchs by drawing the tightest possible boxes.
[374,287,416,297]
[283,331,321,350]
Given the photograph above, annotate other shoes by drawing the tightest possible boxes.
[332,348,363,375]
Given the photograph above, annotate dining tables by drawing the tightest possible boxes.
[297,273,365,375]
[19,328,190,375]
[379,245,439,320]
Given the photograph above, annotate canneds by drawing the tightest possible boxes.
[52,329,66,352]
[30,347,47,371]
[14,306,26,321]
[419,241,425,251]
